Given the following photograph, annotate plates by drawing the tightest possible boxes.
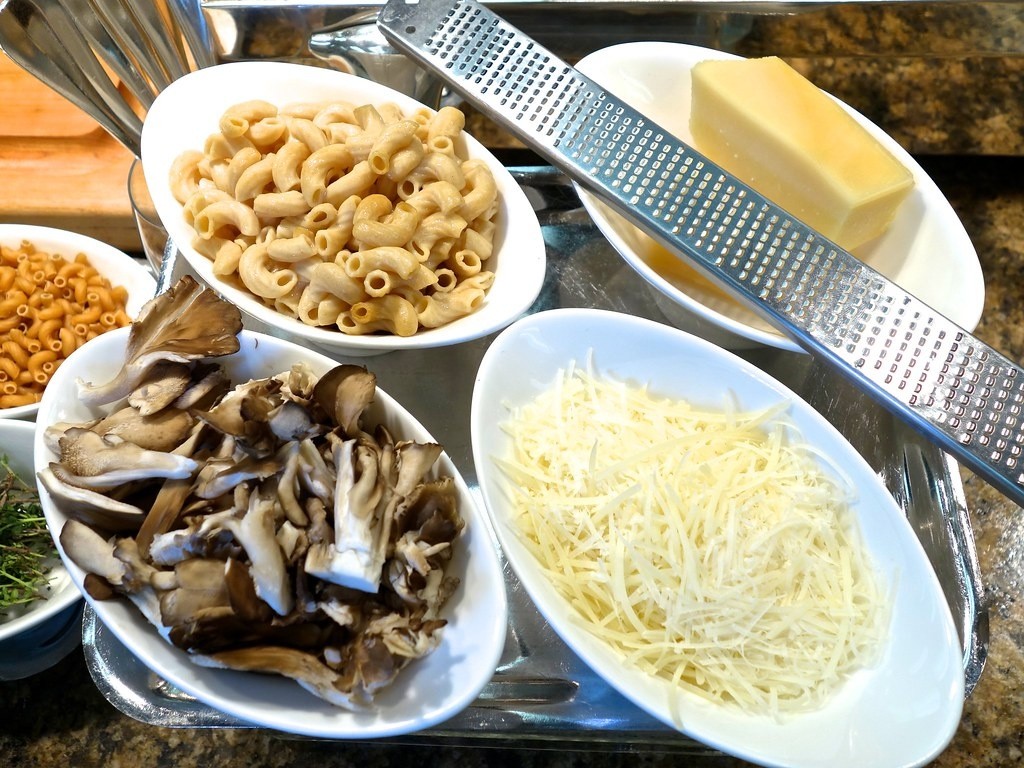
[35,315,509,736]
[0,224,161,422]
[0,415,87,642]
[471,307,966,768]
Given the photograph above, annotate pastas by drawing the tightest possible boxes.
[0,239,134,412]
[167,98,500,338]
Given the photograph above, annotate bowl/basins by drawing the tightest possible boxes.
[566,40,987,359]
[141,63,547,358]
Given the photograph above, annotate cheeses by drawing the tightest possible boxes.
[686,54,913,252]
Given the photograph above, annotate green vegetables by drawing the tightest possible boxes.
[0,452,66,617]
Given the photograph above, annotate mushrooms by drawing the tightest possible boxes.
[36,272,468,719]
